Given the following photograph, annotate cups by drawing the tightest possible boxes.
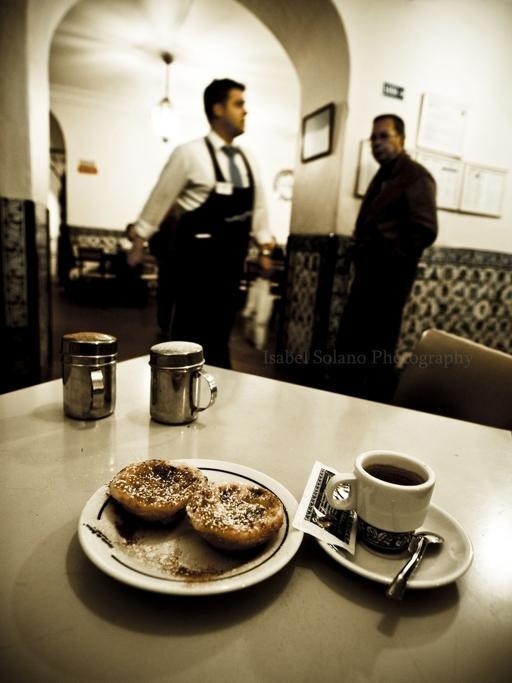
[326,453,435,561]
[57,330,120,417]
[144,340,218,423]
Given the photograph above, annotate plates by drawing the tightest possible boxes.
[72,456,306,597]
[306,487,473,591]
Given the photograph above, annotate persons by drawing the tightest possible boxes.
[330,112,440,402]
[119,223,137,250]
[266,236,285,259]
[128,77,264,371]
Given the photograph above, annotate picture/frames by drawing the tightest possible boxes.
[301,103,333,163]
[352,140,380,198]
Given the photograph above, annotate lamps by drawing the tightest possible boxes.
[154,48,176,145]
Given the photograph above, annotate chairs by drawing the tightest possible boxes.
[391,328,512,434]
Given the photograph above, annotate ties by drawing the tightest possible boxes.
[219,143,243,190]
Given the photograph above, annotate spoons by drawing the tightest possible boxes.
[381,530,446,601]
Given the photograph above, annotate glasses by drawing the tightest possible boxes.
[370,132,400,141]
